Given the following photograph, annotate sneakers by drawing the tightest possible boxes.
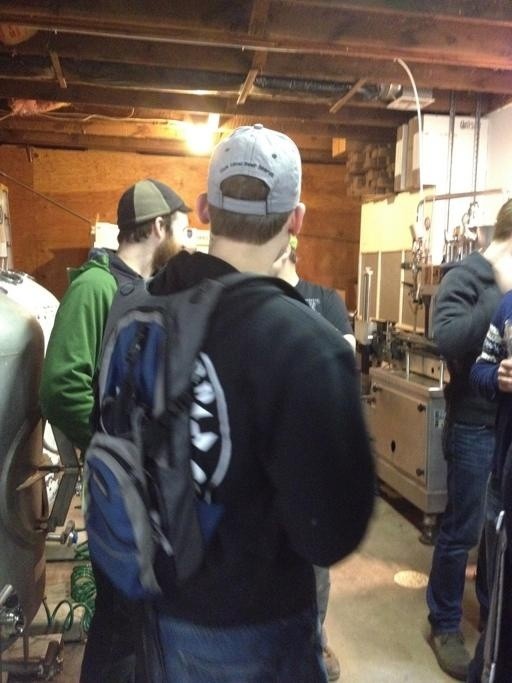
[427,632,471,681]
[321,647,340,680]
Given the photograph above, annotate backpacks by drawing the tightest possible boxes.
[84,273,307,602]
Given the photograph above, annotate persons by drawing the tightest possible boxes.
[427,198,511,682]
[39,123,375,682]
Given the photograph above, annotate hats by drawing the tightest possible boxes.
[207,124,302,215]
[117,178,192,226]
